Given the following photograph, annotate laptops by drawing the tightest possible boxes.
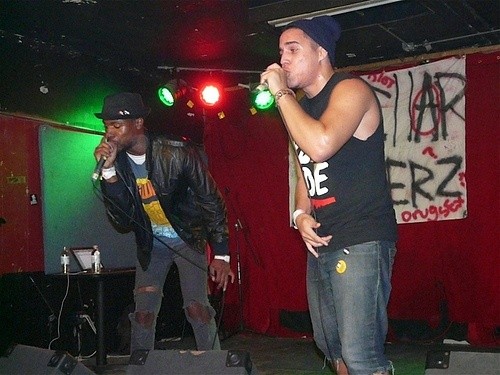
[70,246,121,272]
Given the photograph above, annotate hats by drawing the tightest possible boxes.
[286,15,341,66]
[94,93,151,120]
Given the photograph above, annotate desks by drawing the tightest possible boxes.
[77,269,136,374]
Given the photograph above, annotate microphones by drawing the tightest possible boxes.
[254,82,268,94]
[92,154,106,181]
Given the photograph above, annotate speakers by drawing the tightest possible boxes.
[424,347,500,375]
[0,341,94,375]
[128,349,260,375]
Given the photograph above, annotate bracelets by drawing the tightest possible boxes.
[274,88,297,107]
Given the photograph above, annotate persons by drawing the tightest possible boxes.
[260,15,399,375]
[90,90,235,348]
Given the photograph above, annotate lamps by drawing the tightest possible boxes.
[251,85,275,109]
[158,80,188,107]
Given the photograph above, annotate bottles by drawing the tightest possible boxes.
[91,245,101,274]
[61,246,70,274]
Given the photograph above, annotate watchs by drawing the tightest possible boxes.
[291,208,305,229]
[214,255,231,263]
